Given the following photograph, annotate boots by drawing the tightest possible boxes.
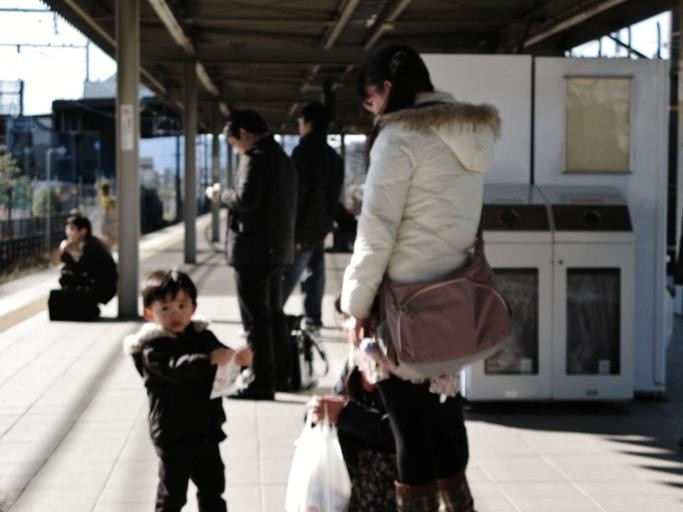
[272,357,302,392]
[227,368,275,401]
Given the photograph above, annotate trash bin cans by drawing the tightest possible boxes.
[461,184,636,405]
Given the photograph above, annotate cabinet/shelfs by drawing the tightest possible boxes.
[459,243,637,402]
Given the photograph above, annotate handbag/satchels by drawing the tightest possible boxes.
[371,239,515,384]
[48,287,100,320]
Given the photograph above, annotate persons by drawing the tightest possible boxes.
[120,269,258,512]
[202,108,302,402]
[56,212,115,305]
[310,291,398,512]
[330,37,504,512]
[281,102,345,332]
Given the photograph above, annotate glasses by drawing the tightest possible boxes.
[362,86,382,113]
